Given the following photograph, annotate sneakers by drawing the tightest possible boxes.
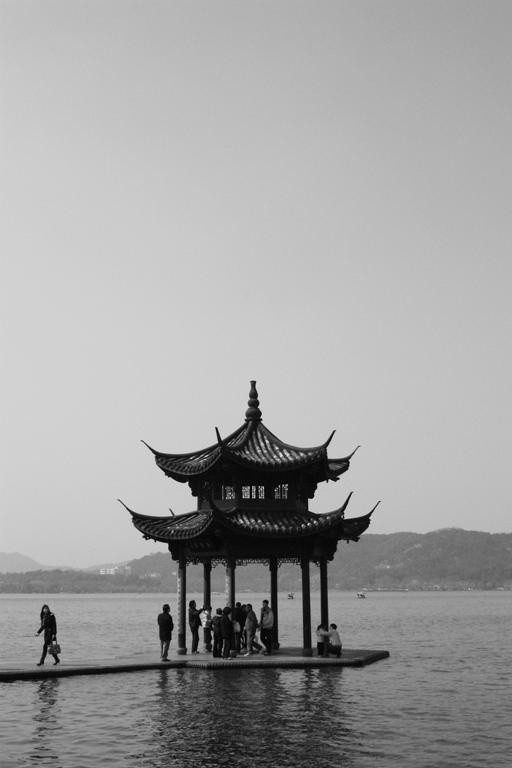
[37,661,44,665]
[54,660,59,665]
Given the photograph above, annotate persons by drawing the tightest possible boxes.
[186,598,279,656]
[157,604,173,662]
[317,623,327,655]
[325,623,343,657]
[34,603,61,664]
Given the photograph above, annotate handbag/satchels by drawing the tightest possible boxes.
[48,644,60,653]
